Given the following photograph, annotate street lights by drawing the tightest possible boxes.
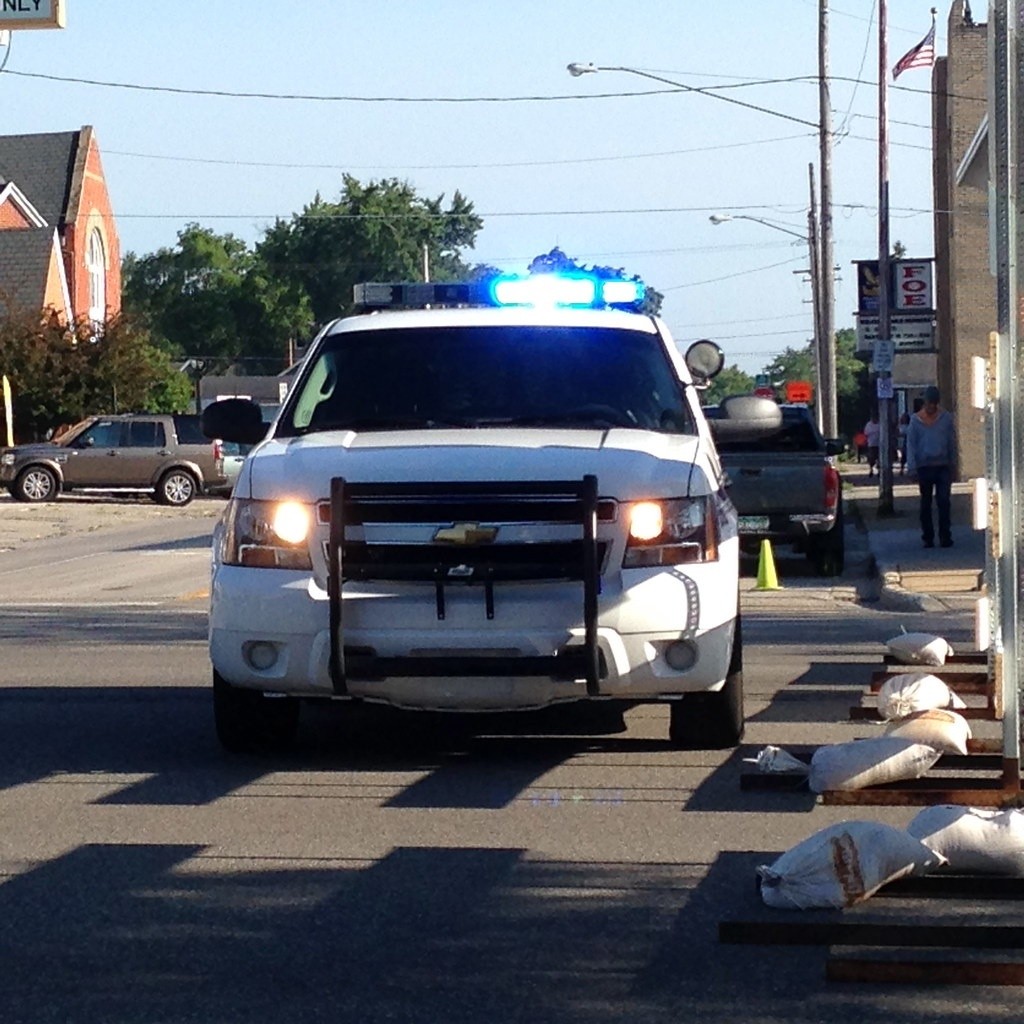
[708,213,832,431]
[567,62,837,436]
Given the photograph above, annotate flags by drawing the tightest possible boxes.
[892,25,934,82]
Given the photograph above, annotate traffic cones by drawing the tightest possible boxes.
[751,538,785,591]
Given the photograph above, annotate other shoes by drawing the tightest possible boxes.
[924,542,934,547]
[941,539,954,548]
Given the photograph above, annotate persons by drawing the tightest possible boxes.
[852,406,916,479]
[905,385,959,549]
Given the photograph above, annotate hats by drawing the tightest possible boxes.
[925,386,940,400]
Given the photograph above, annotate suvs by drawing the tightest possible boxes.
[203,273,784,756]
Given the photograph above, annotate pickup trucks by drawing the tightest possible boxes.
[698,396,846,576]
[0,414,226,508]
